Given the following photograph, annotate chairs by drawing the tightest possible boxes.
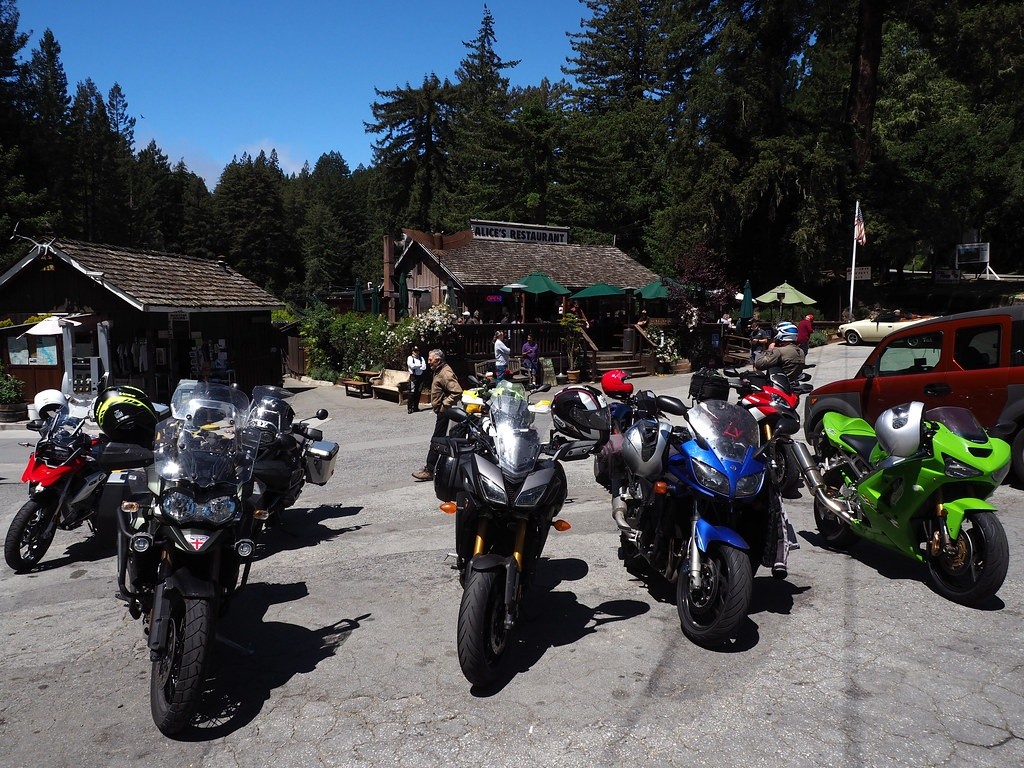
[964,346,983,368]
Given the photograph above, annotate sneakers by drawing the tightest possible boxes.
[412,469,433,482]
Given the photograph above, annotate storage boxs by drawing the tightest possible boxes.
[595,433,624,490]
[434,447,474,502]
[689,375,730,400]
[302,440,340,485]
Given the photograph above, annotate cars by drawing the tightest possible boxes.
[836,310,935,349]
[804,303,1024,487]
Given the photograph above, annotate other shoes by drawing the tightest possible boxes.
[413,408,419,411]
[408,408,413,414]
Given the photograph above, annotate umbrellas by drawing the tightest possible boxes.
[738,279,754,348]
[752,279,818,322]
[634,278,690,318]
[499,271,571,321]
[691,288,757,315]
[570,282,627,346]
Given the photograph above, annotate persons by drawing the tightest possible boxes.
[407,346,426,414]
[798,314,815,358]
[411,350,463,480]
[892,309,905,321]
[755,321,806,396]
[717,313,769,362]
[522,334,540,385]
[458,298,651,352]
[492,331,511,387]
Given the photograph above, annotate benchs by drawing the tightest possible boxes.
[474,357,532,386]
[368,368,410,404]
[343,379,369,399]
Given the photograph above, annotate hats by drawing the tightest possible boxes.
[808,314,814,323]
[412,346,419,351]
[462,311,470,316]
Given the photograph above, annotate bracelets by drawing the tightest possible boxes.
[758,339,759,343]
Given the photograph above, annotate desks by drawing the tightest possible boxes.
[357,370,381,394]
[614,334,624,338]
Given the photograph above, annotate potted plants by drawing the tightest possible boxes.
[0,372,26,423]
[560,312,583,384]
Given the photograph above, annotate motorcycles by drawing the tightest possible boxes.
[718,359,816,492]
[229,384,329,564]
[594,386,666,530]
[792,402,1018,608]
[610,394,800,643]
[3,371,171,576]
[98,379,291,739]
[430,370,613,690]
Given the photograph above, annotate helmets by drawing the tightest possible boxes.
[874,401,925,458]
[773,321,799,342]
[622,419,674,482]
[551,384,612,441]
[33,389,70,419]
[94,384,159,443]
[600,369,633,393]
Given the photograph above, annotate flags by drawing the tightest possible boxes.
[854,201,866,246]
